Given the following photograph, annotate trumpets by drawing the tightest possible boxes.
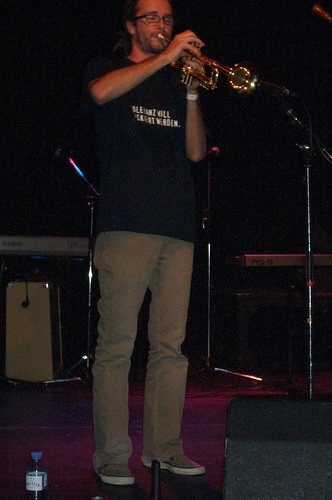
[157,32,261,100]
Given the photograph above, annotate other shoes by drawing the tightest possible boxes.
[142,454,205,476]
[96,464,135,485]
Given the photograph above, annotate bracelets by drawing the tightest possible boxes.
[186,93,199,100]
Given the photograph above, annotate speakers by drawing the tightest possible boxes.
[222,396,332,500]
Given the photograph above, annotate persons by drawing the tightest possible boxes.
[81,0,207,485]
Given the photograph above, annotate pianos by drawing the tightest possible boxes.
[225,253,332,268]
[0,235,91,258]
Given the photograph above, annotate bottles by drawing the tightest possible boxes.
[25,451,48,500]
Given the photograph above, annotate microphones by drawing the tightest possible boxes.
[256,80,296,97]
[204,147,220,159]
[55,149,72,160]
[312,4,332,24]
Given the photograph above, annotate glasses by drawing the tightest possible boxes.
[134,13,175,25]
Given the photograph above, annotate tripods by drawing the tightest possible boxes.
[44,161,94,386]
[185,95,332,402]
